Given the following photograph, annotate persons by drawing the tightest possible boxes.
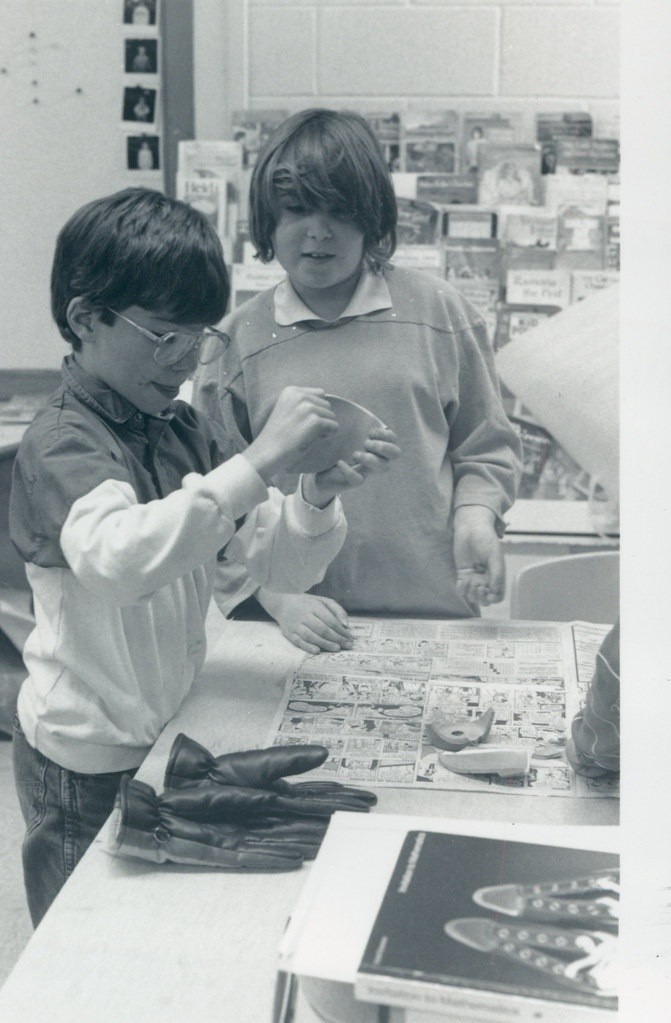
[6,186,403,933]
[191,107,525,656]
[466,126,488,172]
[233,130,249,167]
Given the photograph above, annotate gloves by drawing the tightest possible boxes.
[107,774,371,868]
[164,734,377,806]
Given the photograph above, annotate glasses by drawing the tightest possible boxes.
[107,307,231,368]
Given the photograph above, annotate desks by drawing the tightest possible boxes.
[0,615,618,1023]
